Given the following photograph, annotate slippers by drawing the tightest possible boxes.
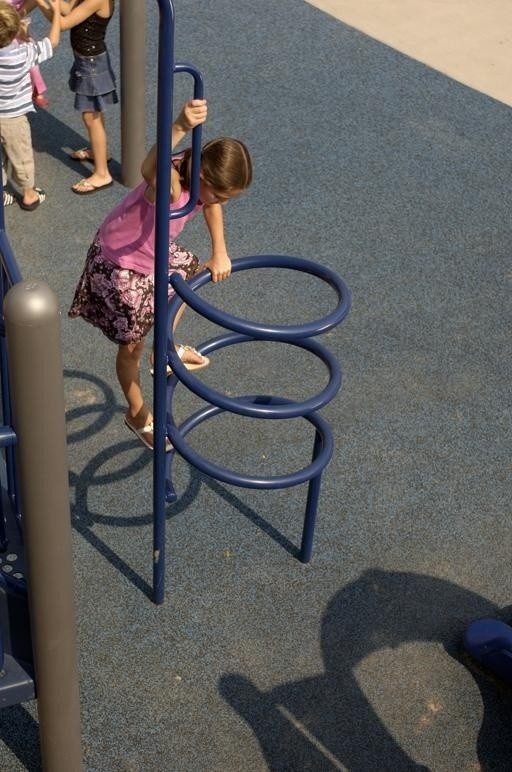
[2,187,46,211]
[71,147,111,163]
[124,418,174,452]
[150,343,210,376]
[72,179,112,194]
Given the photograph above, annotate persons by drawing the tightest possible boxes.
[69,97,253,452]
[0,0,61,210]
[32,0,118,194]
[0,0,49,107]
